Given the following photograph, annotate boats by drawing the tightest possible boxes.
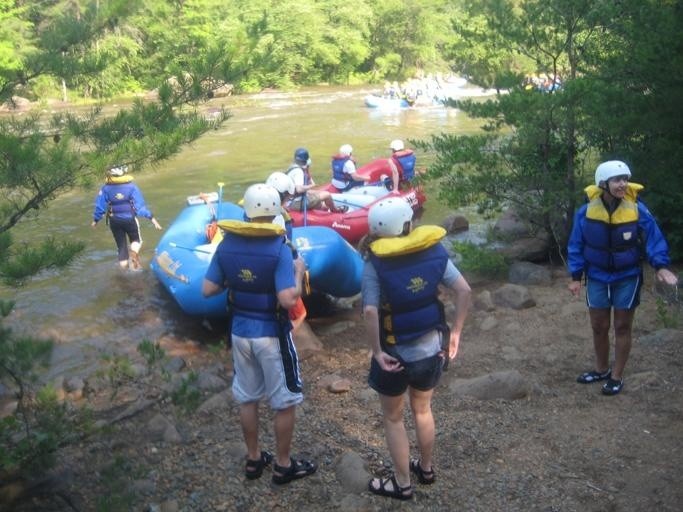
[364,74,565,109]
[284,153,429,240]
[151,200,365,317]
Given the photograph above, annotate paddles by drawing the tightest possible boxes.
[302,159,312,226]
[366,166,428,186]
[211,182,224,244]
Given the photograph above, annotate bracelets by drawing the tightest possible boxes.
[295,270,305,278]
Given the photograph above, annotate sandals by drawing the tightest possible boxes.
[272,458,318,484]
[410,460,435,484]
[246,452,272,479]
[368,475,413,500]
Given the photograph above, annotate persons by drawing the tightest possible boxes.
[358,195,474,501]
[200,183,318,487]
[266,170,309,333]
[370,59,568,111]
[88,158,165,275]
[330,142,372,192]
[283,146,349,214]
[379,139,418,196]
[566,159,679,396]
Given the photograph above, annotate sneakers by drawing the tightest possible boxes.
[601,378,623,395]
[330,206,348,213]
[577,368,611,384]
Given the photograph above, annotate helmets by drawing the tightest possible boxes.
[109,163,129,176]
[295,148,308,163]
[340,144,353,155]
[594,161,632,187]
[390,140,404,151]
[266,172,295,196]
[244,184,281,219]
[368,198,416,238]
